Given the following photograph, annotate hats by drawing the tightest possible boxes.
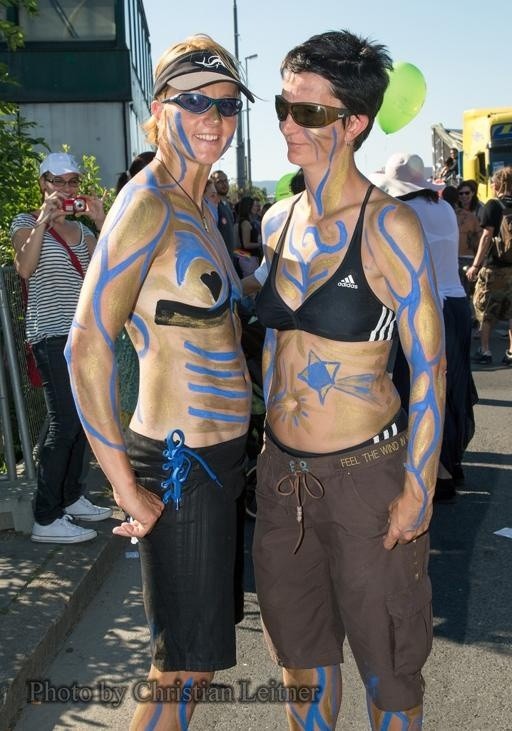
[362,152,444,198]
[39,151,82,180]
[152,49,255,104]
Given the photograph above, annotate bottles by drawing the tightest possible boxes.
[461,266,478,282]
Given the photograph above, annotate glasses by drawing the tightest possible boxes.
[274,94,359,128]
[160,92,244,117]
[44,177,82,188]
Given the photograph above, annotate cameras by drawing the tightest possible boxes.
[63,197,87,213]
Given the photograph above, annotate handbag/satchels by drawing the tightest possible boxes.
[24,335,46,390]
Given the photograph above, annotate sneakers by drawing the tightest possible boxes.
[470,347,492,366]
[61,495,113,524]
[30,518,97,545]
[470,326,482,338]
[500,333,509,341]
[502,349,512,367]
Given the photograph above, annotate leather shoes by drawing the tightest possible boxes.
[431,459,456,505]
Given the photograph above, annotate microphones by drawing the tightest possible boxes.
[463,265,477,280]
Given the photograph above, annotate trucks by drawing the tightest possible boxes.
[431,107,510,222]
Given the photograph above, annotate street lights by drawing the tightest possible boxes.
[244,52,259,185]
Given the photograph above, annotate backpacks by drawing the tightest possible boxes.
[490,196,512,265]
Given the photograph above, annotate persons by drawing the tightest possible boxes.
[251,29,448,730]
[62,32,252,731]
